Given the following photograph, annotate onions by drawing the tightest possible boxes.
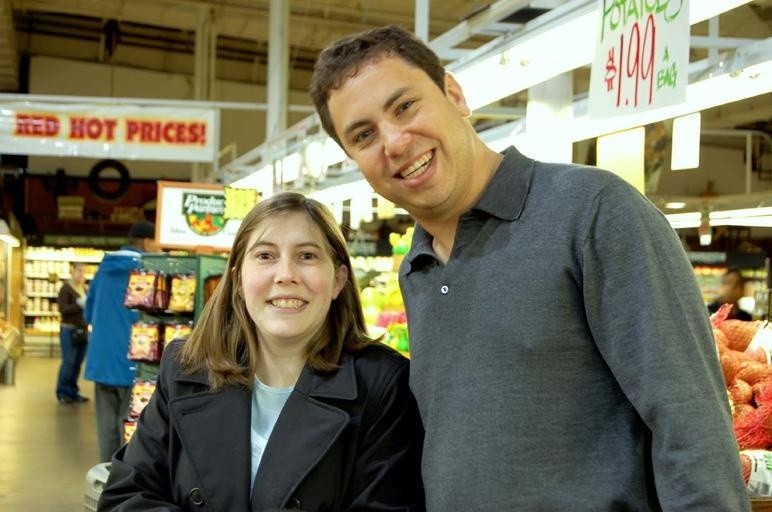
[712,321,772,482]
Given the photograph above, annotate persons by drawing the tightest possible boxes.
[81,217,169,468]
[303,21,753,512]
[56,263,92,405]
[92,188,428,512]
[704,263,754,322]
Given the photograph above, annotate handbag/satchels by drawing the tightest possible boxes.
[71,328,87,347]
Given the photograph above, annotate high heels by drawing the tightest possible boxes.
[56,390,88,404]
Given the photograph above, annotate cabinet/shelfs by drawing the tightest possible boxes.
[124,252,229,385]
[24,251,103,357]
